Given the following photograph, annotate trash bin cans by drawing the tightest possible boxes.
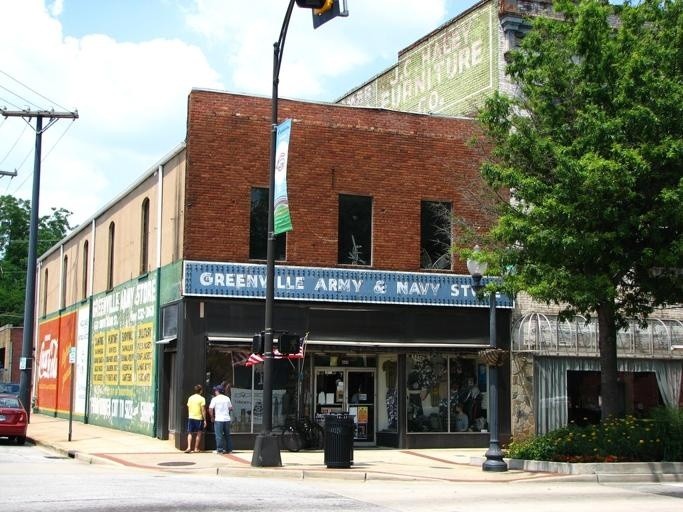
[324,414,356,468]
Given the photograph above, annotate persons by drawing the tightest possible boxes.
[406,380,427,420]
[207,384,236,455]
[451,403,470,432]
[181,384,208,454]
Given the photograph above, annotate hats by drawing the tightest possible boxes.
[212,385,225,392]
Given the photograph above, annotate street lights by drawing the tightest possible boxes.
[467,244,524,472]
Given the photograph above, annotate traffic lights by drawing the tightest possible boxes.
[253,332,300,354]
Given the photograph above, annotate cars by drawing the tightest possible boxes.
[0,394,28,445]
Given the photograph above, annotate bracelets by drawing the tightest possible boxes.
[203,416,206,421]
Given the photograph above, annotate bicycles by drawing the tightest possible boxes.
[282,415,325,452]
